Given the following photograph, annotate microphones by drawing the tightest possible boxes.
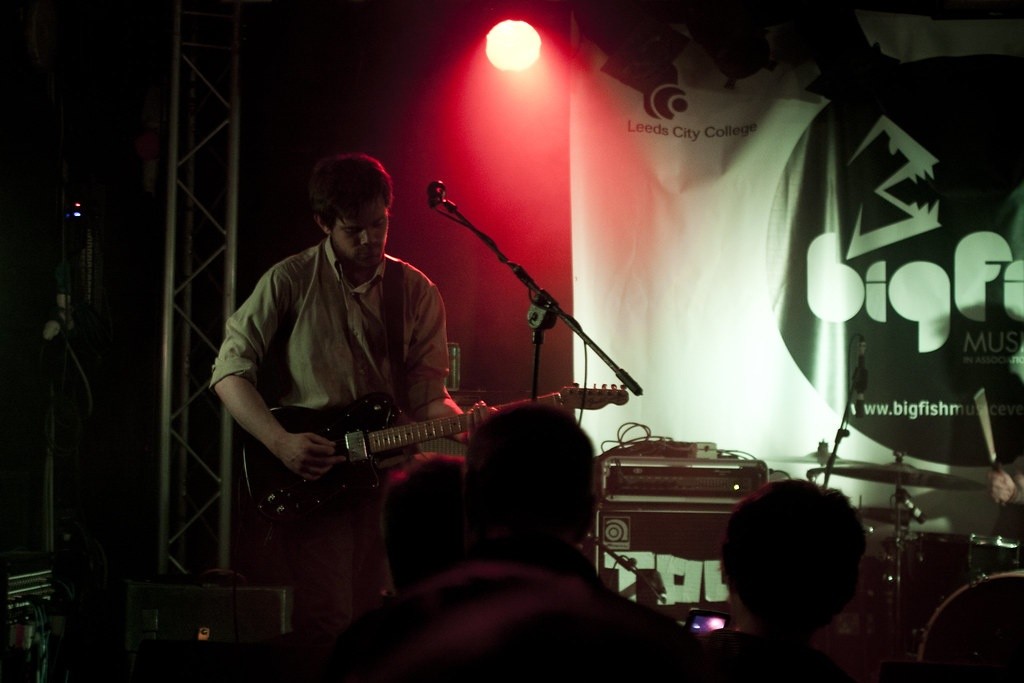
[427,180,446,209]
[900,493,926,525]
[852,338,867,417]
[647,568,667,604]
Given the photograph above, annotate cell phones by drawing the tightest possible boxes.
[684,607,732,633]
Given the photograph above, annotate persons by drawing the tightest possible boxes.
[210,152,498,644]
[324,403,694,683]
[704,479,867,683]
[989,464,1024,504]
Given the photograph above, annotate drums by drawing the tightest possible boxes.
[891,532,1024,656]
[916,569,1024,664]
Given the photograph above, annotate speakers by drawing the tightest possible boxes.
[125,584,298,683]
[591,500,741,630]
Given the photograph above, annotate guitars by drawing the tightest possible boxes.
[242,379,631,525]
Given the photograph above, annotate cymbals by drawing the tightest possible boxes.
[849,504,941,524]
[816,462,986,491]
[761,452,881,465]
